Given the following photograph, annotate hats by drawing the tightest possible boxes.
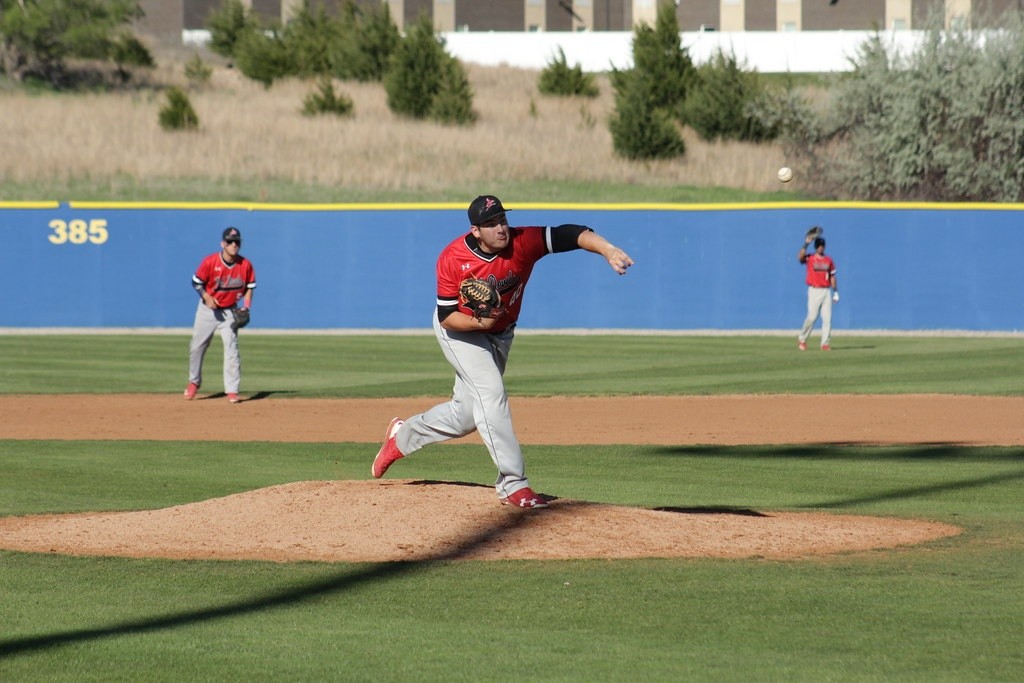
[815,238,826,248]
[223,228,241,243]
[468,196,514,224]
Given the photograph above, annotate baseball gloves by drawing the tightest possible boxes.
[459,271,508,321]
[229,306,250,330]
[806,226,822,241]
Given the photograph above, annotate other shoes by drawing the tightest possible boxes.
[227,393,241,402]
[820,345,832,350]
[184,382,198,399]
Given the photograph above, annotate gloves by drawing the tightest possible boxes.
[832,291,839,302]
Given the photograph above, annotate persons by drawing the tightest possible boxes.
[183,227,256,403]
[797,226,840,351]
[371,193,635,510]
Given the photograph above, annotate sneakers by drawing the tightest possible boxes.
[371,418,405,478]
[501,487,549,509]
[799,342,807,350]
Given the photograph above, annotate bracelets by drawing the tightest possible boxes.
[803,242,810,249]
[833,288,837,291]
[243,300,251,308]
